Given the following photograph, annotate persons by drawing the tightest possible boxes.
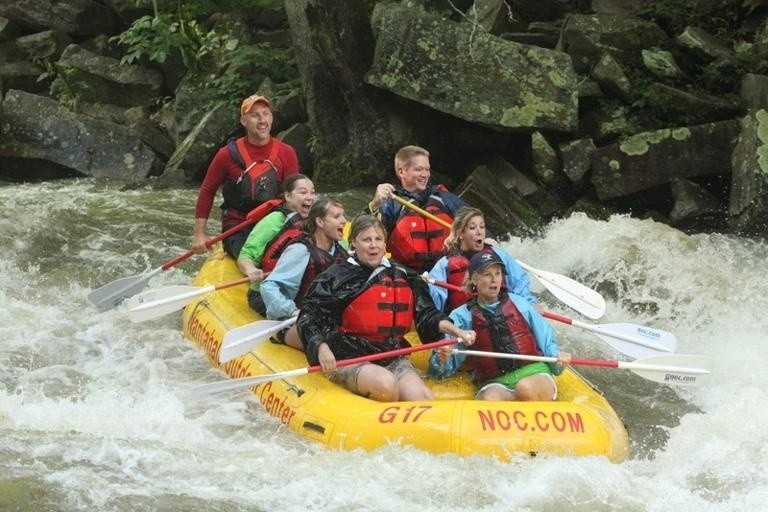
[422,206,545,315]
[192,94,300,260]
[238,173,348,319]
[362,145,498,275]
[296,214,477,402]
[427,251,572,401]
[259,198,353,351]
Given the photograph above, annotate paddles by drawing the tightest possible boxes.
[390,195,605,321]
[197,337,464,400]
[427,277,677,361]
[454,352,713,384]
[220,316,298,362]
[126,271,273,324]
[89,212,262,312]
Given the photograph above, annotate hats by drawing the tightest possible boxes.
[241,95,270,114]
[469,253,504,276]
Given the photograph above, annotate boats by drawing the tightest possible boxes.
[177,244,634,464]
